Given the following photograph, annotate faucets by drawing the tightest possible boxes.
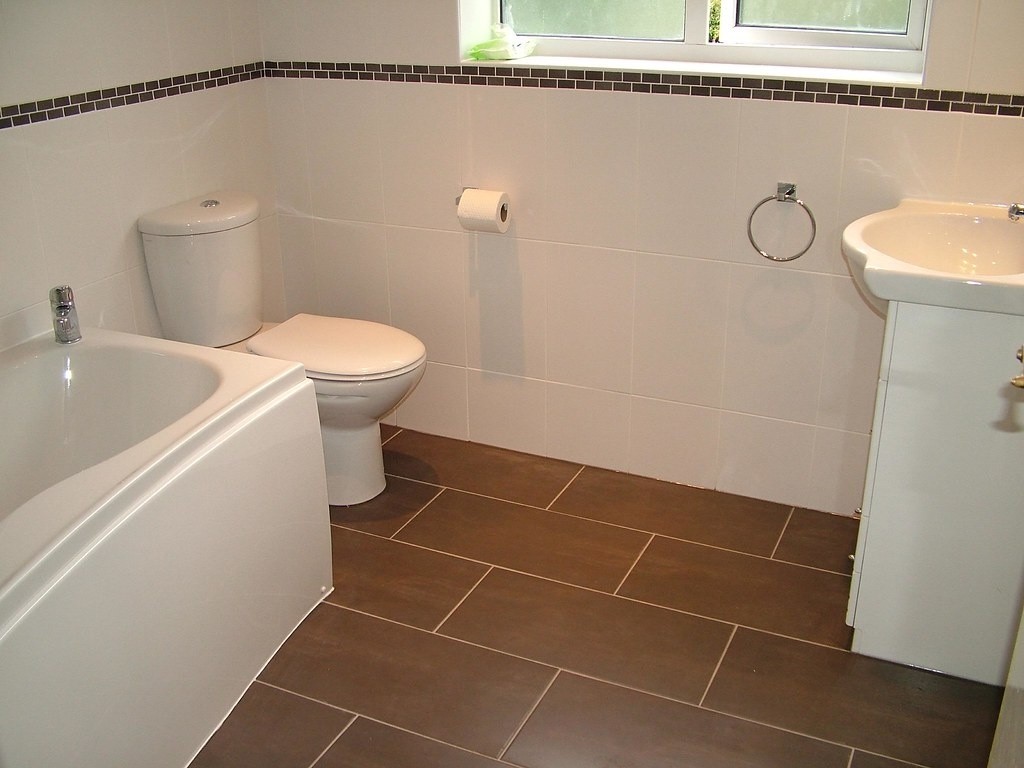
[48,284,82,347]
[1009,200,1023,221]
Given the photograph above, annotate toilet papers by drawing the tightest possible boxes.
[456,188,511,234]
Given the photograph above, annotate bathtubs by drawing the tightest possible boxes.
[0,327,335,766]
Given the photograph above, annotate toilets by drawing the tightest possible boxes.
[138,191,428,506]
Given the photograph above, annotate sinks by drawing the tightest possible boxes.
[841,197,1023,322]
